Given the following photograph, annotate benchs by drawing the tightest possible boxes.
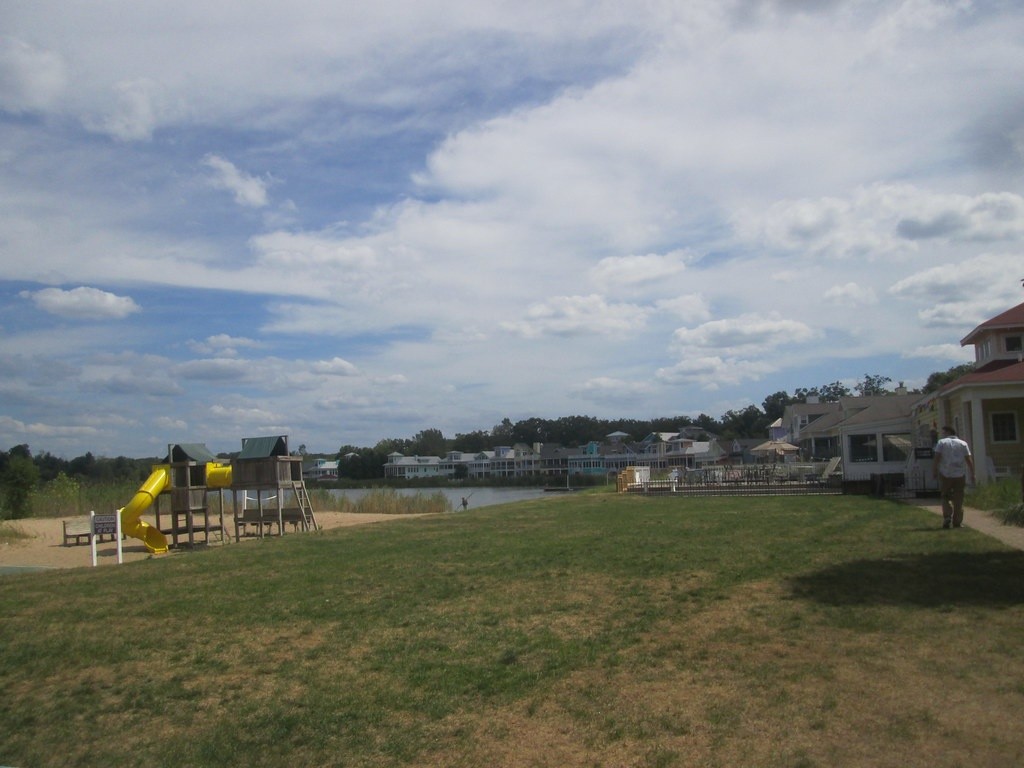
[238,506,310,537]
[62,517,125,547]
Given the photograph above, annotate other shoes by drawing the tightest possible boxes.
[954,523,965,528]
[943,517,951,528]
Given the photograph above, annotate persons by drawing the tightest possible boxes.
[933,425,978,528]
[461,497,468,511]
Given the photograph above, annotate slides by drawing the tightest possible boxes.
[120,468,169,555]
